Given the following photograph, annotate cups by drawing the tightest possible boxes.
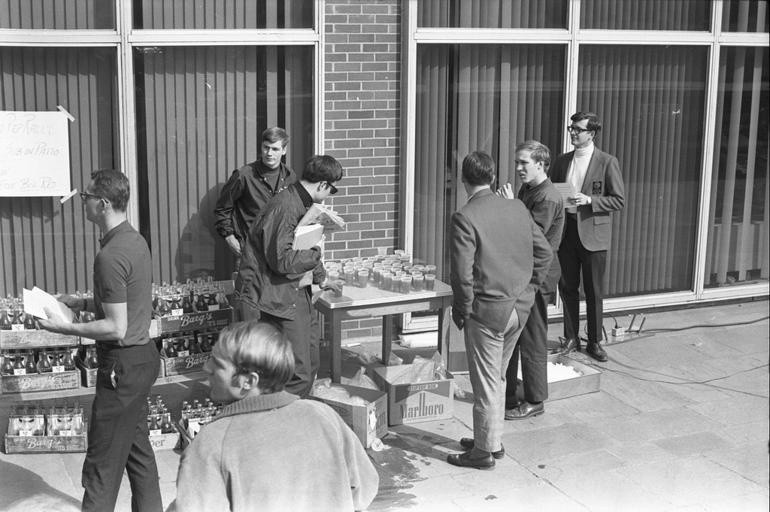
[325,253,436,295]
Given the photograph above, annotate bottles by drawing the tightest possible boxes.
[1,350,75,374]
[78,347,99,369]
[1,293,35,330]
[180,396,222,432]
[8,398,83,435]
[159,327,220,357]
[152,276,228,316]
[147,395,173,432]
[70,290,95,321]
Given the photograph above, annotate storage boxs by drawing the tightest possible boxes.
[310,361,454,449]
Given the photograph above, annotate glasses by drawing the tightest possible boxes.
[79,192,109,203]
[320,182,337,194]
[567,127,591,134]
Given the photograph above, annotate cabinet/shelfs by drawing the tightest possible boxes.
[1,298,235,402]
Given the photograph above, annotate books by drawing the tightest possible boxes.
[292,203,346,251]
[21,284,77,327]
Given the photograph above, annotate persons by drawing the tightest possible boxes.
[31,167,166,512]
[212,126,302,269]
[161,320,381,512]
[550,111,629,364]
[495,138,566,422]
[446,149,555,473]
[230,155,346,400]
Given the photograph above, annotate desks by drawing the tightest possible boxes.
[314,277,453,387]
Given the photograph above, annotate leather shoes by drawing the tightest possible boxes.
[551,337,581,356]
[505,401,544,419]
[461,438,504,459]
[586,340,608,362]
[506,395,520,409]
[447,452,496,470]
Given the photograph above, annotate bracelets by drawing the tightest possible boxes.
[585,195,592,206]
[82,298,88,312]
